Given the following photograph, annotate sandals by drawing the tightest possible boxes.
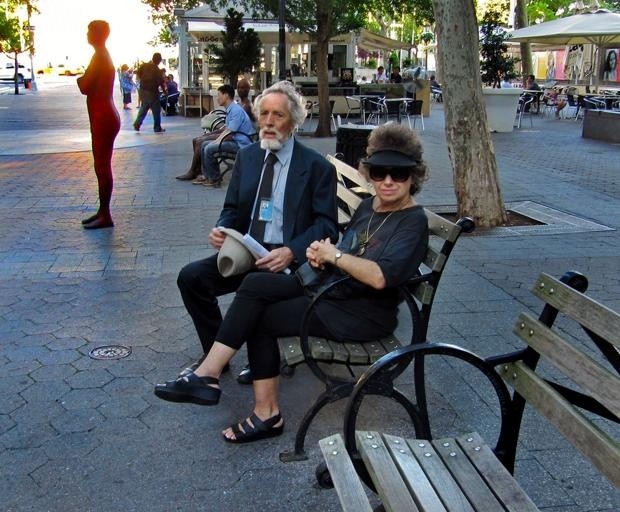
[152,372,225,407]
[222,412,286,444]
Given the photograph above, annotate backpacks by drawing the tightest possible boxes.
[295,227,362,301]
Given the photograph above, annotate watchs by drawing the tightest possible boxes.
[334,251,345,266]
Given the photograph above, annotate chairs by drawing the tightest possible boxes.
[428,84,620,130]
[163,91,180,117]
[306,92,425,136]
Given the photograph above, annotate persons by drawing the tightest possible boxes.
[178,82,339,386]
[542,81,567,118]
[520,75,541,113]
[358,65,443,103]
[76,20,123,230]
[155,123,429,442]
[501,76,513,89]
[118,53,178,132]
[603,51,618,81]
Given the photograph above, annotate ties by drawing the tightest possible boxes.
[249,152,279,247]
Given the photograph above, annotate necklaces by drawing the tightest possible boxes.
[358,203,411,256]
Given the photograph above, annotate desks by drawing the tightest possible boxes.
[181,86,203,119]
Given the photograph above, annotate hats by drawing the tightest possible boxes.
[361,149,419,167]
[216,226,261,279]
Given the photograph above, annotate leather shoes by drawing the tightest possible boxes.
[176,353,231,377]
[237,362,253,385]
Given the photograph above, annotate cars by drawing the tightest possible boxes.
[37,60,85,77]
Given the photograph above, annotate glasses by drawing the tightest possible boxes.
[368,164,412,182]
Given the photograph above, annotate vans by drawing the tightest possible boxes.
[0,56,32,84]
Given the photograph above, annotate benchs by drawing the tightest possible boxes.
[317,268,620,508]
[274,154,477,463]
[205,104,265,187]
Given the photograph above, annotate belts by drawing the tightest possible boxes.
[261,243,284,252]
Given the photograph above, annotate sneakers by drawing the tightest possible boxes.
[154,126,165,132]
[123,105,140,110]
[192,175,223,187]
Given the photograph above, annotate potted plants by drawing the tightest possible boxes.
[477,10,523,133]
